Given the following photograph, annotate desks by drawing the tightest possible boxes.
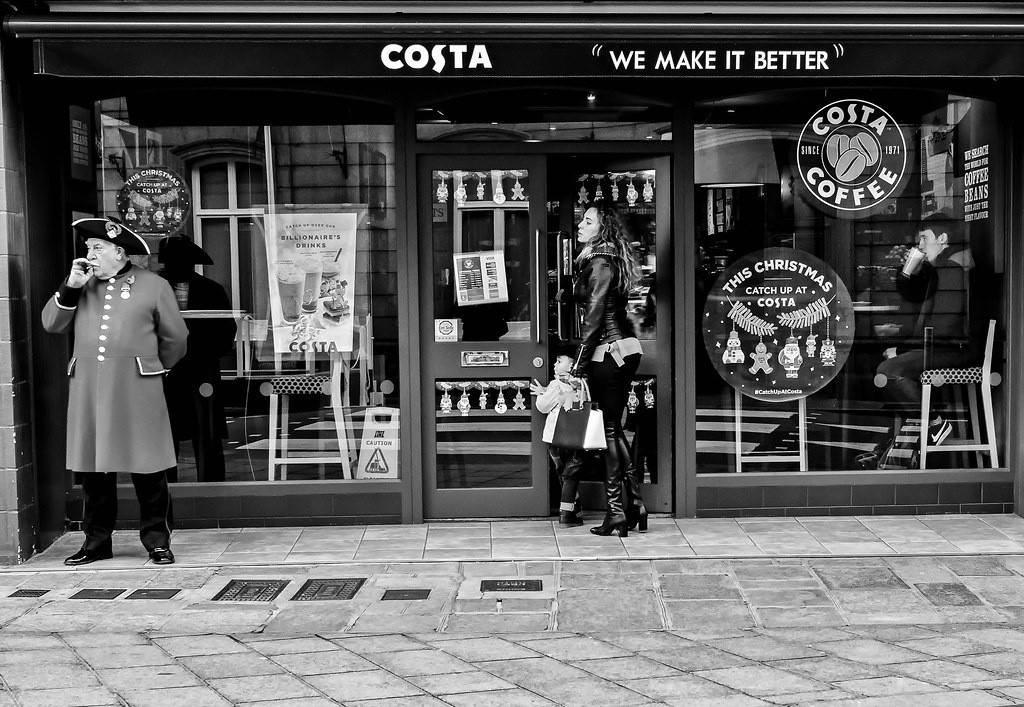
[840,304,902,469]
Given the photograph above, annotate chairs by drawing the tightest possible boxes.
[920,319,1000,470]
[734,387,808,473]
[267,324,367,480]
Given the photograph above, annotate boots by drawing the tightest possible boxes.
[614,430,648,531]
[590,422,628,537]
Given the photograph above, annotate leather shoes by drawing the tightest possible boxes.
[65,548,113,565]
[149,547,175,564]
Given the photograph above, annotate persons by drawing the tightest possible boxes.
[155,234,239,482]
[42,217,189,566]
[877,214,968,446]
[530,350,589,526]
[569,199,648,536]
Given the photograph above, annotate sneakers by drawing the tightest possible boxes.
[559,510,583,527]
[574,498,584,517]
[917,418,953,454]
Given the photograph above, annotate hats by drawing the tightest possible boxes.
[551,344,577,359]
[71,216,151,255]
[159,233,215,268]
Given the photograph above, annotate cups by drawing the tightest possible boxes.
[900,248,925,278]
[320,259,343,297]
[295,263,323,313]
[277,272,306,324]
[175,282,190,309]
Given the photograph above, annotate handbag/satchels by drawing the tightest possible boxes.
[542,374,608,451]
